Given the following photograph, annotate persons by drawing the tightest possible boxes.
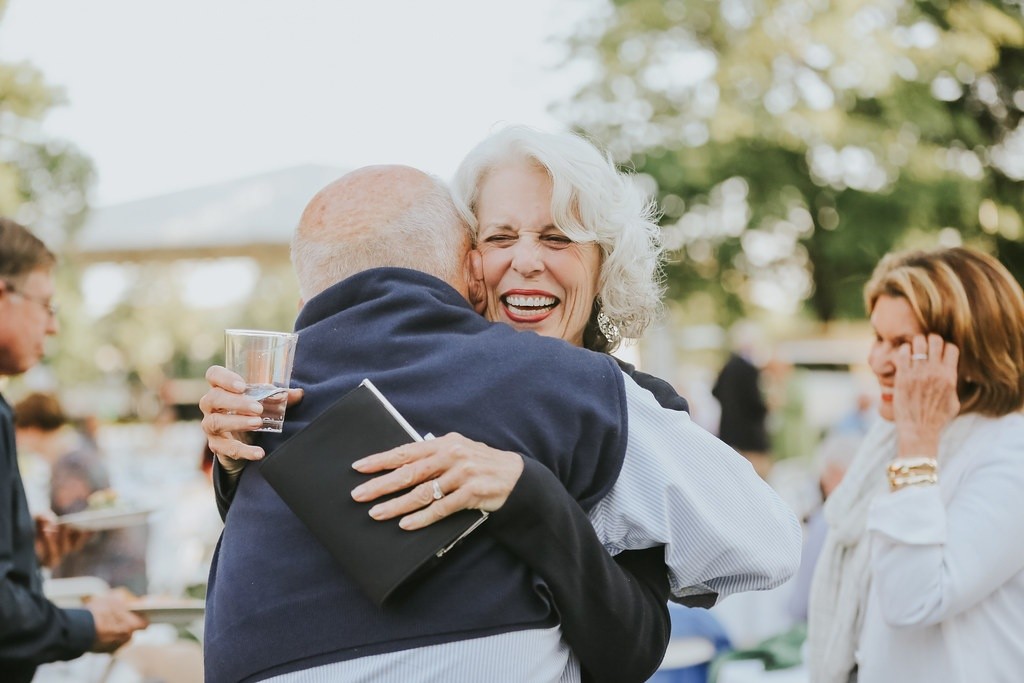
[0,120,1024,683]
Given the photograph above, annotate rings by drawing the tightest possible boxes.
[912,353,928,362]
[432,479,444,499]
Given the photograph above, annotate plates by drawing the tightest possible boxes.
[122,598,205,624]
[50,507,155,531]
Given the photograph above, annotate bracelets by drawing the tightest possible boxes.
[888,473,940,490]
[886,455,940,476]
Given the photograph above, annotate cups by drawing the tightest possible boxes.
[223,328,299,434]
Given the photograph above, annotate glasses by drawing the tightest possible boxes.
[5,279,61,315]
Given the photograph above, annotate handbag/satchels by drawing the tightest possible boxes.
[257,378,490,609]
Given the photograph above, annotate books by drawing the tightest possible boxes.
[259,378,489,611]
